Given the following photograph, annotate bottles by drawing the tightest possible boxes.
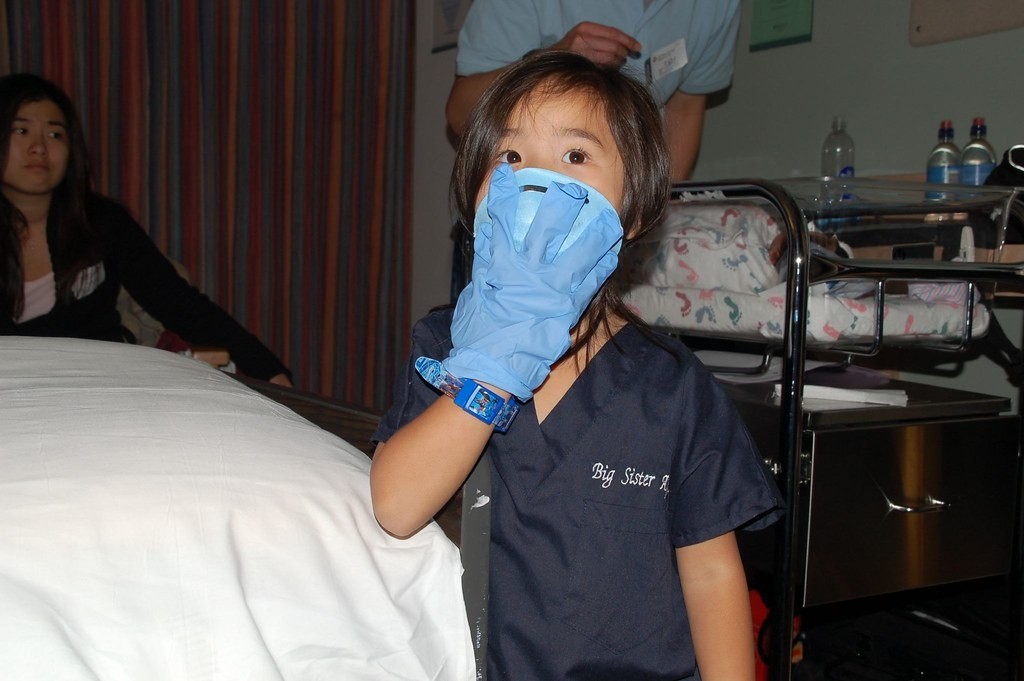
[822,116,855,203]
[961,118,996,198]
[925,119,962,203]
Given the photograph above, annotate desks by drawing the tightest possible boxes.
[771,174,1023,258]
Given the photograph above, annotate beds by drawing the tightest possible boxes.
[0,334,477,681]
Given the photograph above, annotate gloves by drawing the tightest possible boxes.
[441,162,624,402]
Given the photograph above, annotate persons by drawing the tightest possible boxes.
[369,51,778,681]
[446,0,742,306]
[0,75,294,389]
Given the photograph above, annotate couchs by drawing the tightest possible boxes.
[116,258,236,373]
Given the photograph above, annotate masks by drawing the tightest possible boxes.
[460,167,650,262]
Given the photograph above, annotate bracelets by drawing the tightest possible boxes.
[523,48,543,60]
[415,356,521,434]
